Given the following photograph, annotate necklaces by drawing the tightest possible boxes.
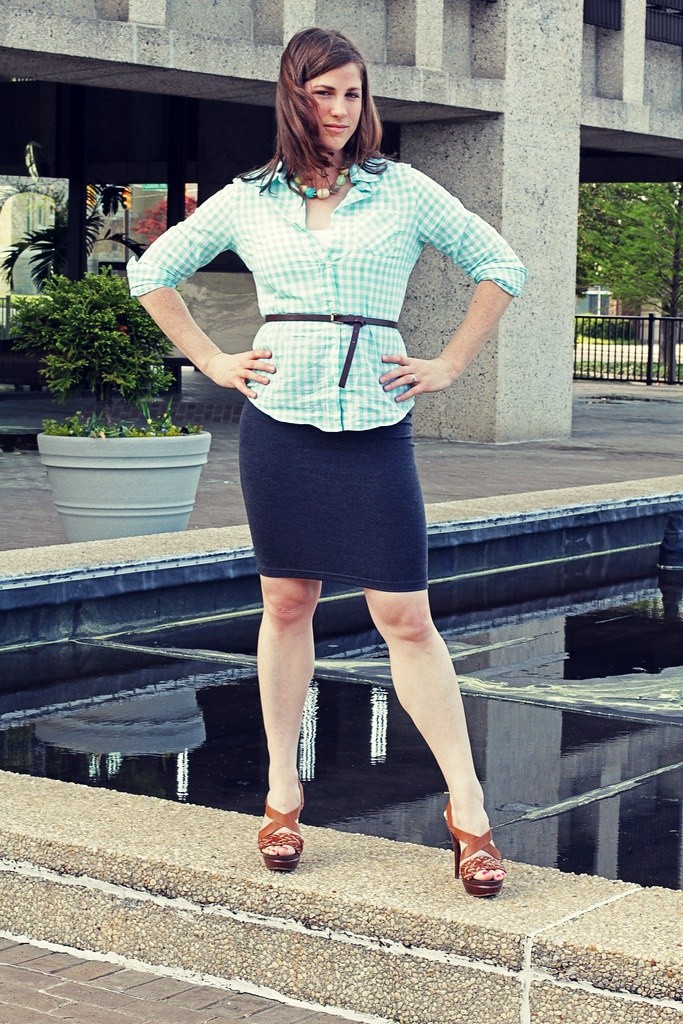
[290,164,349,200]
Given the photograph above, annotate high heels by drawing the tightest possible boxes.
[443,798,508,897]
[258,781,304,870]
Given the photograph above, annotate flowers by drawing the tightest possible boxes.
[7,266,211,438]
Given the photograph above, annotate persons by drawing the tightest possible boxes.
[129,26,527,899]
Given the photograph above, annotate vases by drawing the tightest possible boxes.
[37,431,212,543]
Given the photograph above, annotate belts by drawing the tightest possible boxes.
[265,314,399,388]
